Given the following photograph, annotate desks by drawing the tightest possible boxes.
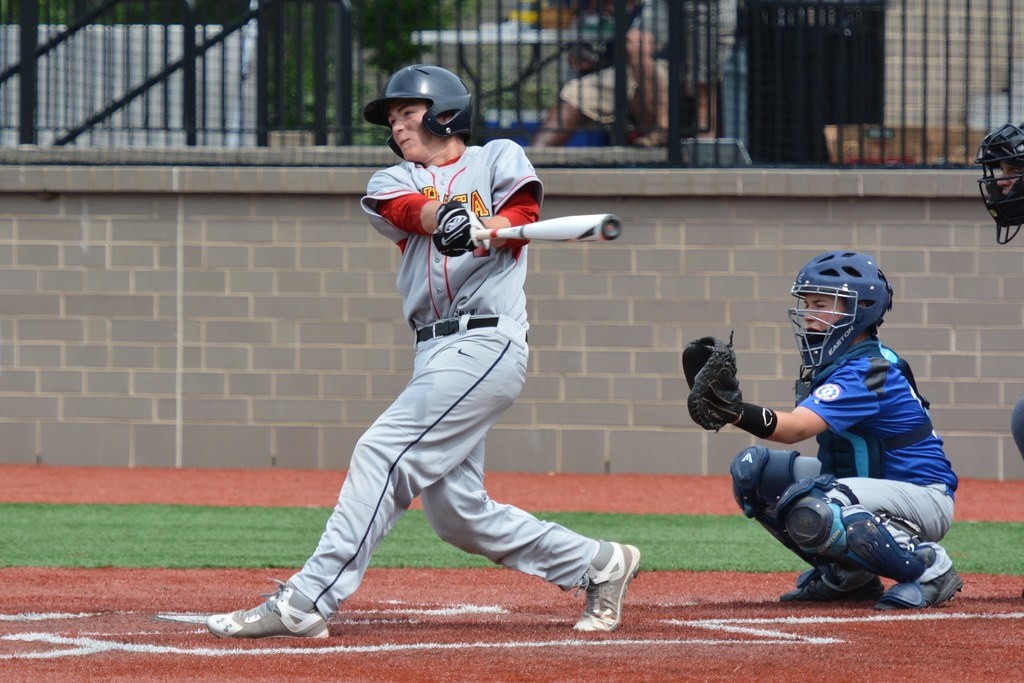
[410,22,617,139]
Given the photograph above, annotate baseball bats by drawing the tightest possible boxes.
[471,215,622,244]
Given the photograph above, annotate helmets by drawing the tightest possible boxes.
[974,122,1024,245]
[364,65,472,159]
[787,249,893,369]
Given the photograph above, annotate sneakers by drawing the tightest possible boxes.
[780,575,884,602]
[573,540,642,630]
[873,565,962,611]
[207,581,329,638]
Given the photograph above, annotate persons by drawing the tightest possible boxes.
[681,249,963,611]
[205,62,642,639]
[974,123,1024,461]
[523,0,739,149]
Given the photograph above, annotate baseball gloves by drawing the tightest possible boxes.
[680,333,745,431]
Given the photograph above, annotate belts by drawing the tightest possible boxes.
[416,316,529,342]
